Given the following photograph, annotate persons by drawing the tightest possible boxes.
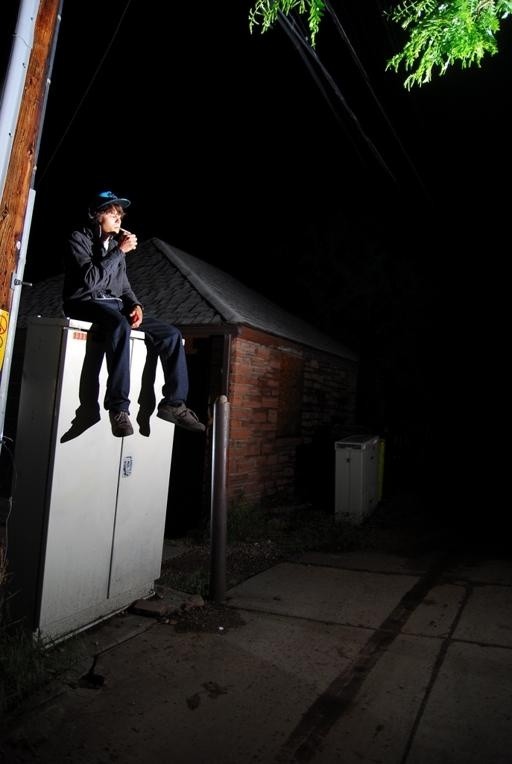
[62,189,209,437]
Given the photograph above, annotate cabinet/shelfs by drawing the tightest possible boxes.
[6,316,179,651]
[335,434,379,526]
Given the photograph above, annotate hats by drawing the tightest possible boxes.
[89,190,131,213]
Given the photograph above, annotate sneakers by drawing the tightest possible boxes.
[106,406,135,438]
[157,399,206,434]
